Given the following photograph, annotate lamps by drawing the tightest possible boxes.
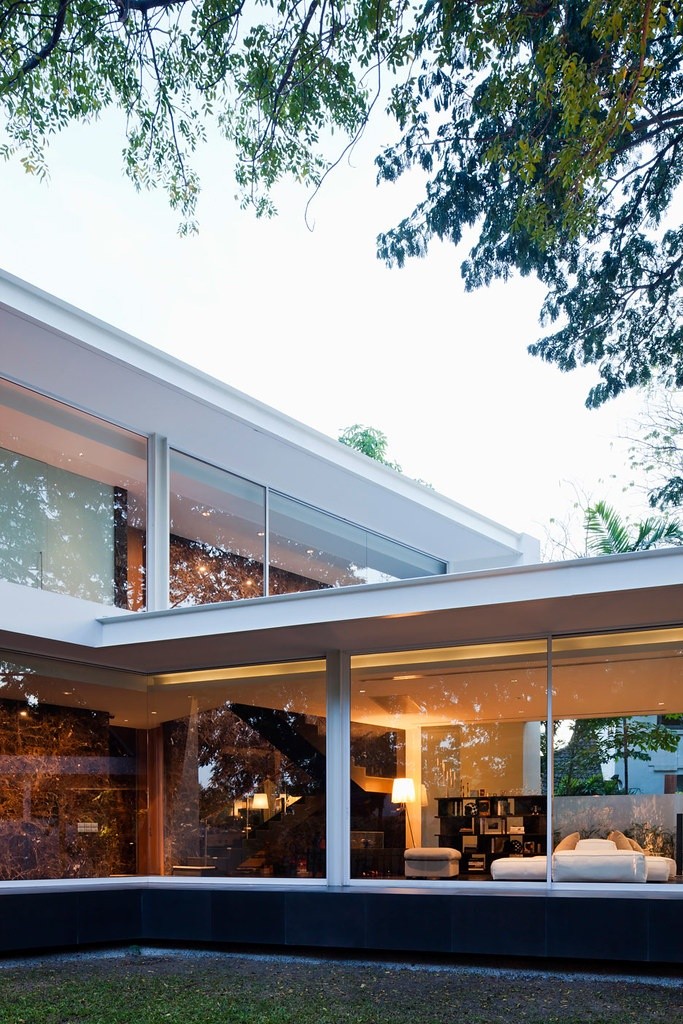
[253,793,269,824]
[389,778,414,816]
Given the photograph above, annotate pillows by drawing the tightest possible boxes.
[554,832,580,852]
[607,830,644,853]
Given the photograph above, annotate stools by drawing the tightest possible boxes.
[404,847,461,880]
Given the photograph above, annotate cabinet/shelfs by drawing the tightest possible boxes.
[434,795,547,874]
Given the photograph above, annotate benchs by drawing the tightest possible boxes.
[490,839,676,882]
